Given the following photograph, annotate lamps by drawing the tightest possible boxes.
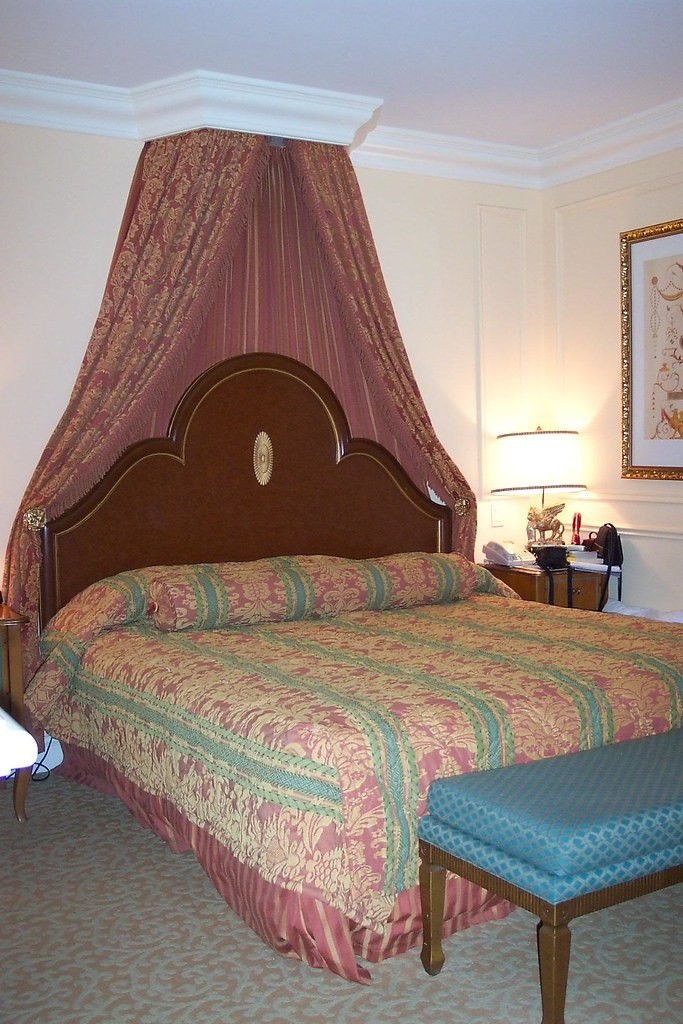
[491,426,587,540]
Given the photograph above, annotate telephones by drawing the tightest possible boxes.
[482,541,536,566]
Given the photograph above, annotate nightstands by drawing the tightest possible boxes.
[477,557,608,613]
[0,603,32,822]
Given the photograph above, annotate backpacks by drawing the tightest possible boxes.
[594,522,623,566]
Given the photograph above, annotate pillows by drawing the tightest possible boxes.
[146,552,479,632]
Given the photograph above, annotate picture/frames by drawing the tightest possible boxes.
[619,218,683,482]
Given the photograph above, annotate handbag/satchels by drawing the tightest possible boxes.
[536,546,567,569]
[582,532,599,552]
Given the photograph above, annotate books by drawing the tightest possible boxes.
[567,549,621,572]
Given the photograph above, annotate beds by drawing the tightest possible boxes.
[0,351,683,986]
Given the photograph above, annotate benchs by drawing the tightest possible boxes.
[417,729,683,1024]
